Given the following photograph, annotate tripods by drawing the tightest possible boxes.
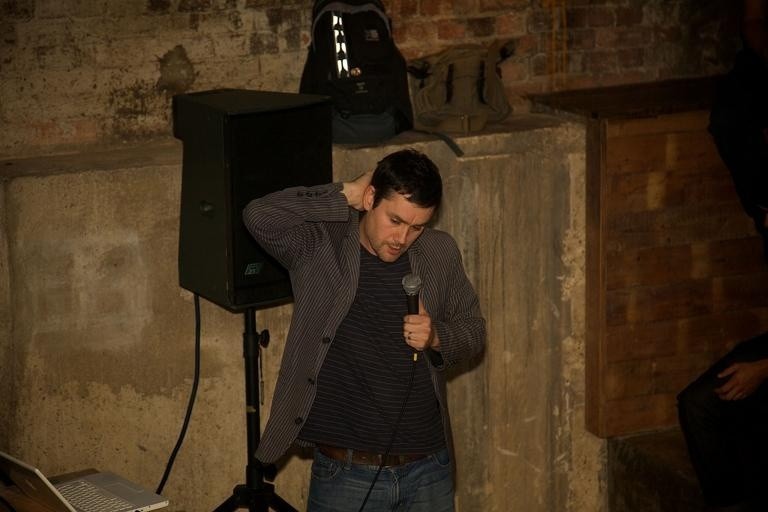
[210,310,298,512]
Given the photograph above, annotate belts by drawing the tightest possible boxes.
[320,446,425,467]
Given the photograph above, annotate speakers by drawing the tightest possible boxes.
[170,87,334,314]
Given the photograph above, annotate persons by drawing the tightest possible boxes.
[676,333,768,510]
[242,148,488,511]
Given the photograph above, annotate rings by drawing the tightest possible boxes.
[407,332,412,339]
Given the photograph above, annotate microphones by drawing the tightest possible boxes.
[399,273,425,363]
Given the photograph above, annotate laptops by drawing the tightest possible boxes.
[1,450,169,512]
